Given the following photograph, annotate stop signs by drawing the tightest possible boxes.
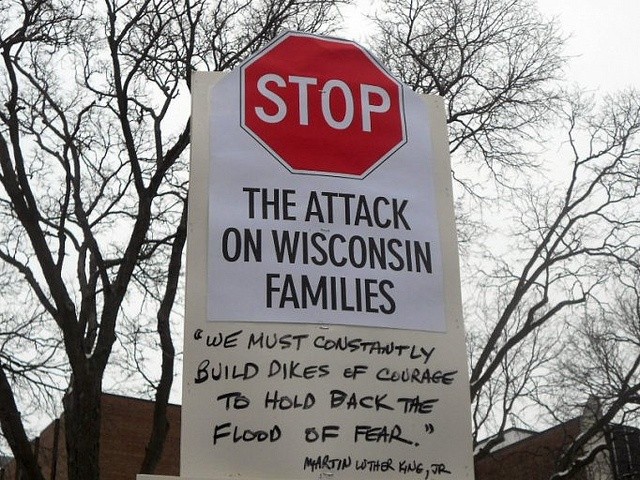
[239,30,408,180]
